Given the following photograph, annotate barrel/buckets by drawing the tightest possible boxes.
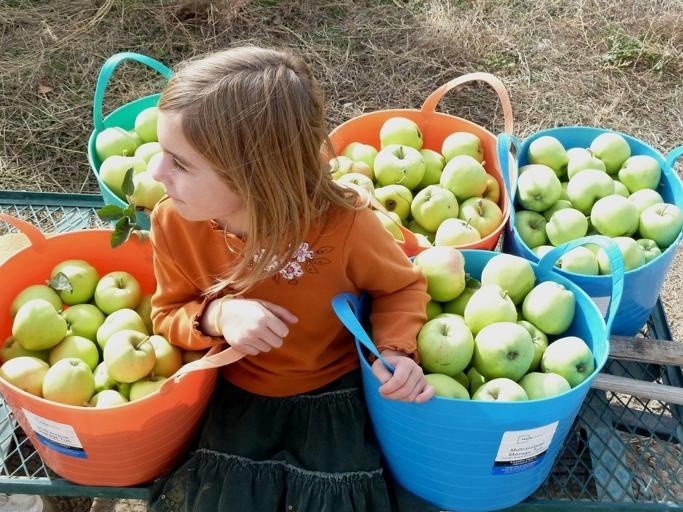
[329,234,625,512]
[87,52,172,230]
[1,213,243,512]
[495,124,683,337]
[318,73,517,254]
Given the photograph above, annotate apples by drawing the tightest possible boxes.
[328,116,504,245]
[0,259,210,409]
[96,105,168,212]
[515,132,682,276]
[412,246,596,400]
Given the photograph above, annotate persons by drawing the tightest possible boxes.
[146,46,435,512]
